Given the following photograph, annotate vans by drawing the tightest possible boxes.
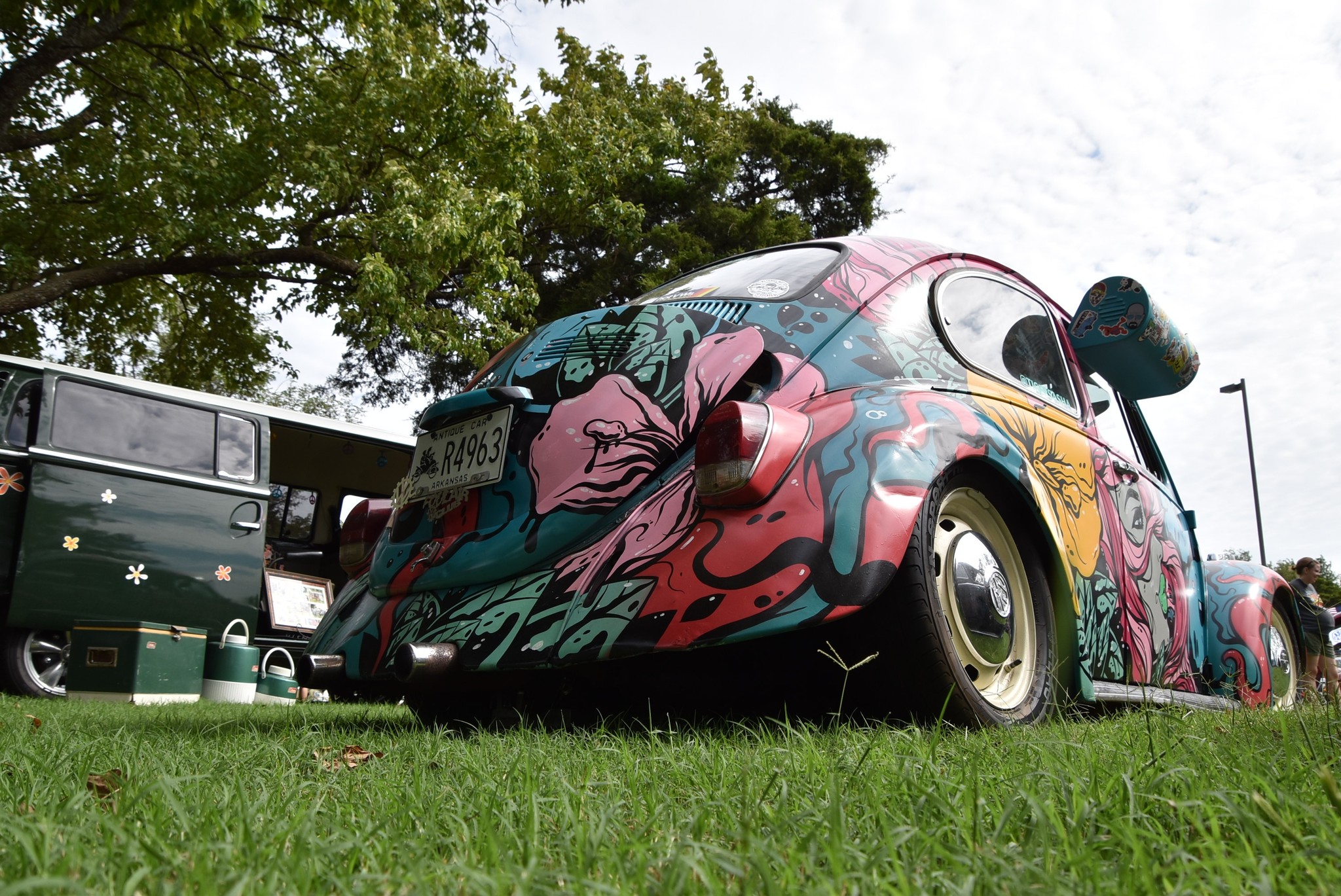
[0,352,428,700]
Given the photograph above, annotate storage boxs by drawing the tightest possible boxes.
[65,619,208,706]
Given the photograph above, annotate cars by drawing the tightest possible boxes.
[300,236,1305,732]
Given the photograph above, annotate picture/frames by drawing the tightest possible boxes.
[264,567,335,635]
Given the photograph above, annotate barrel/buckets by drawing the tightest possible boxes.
[199,618,260,711]
[253,648,298,709]
